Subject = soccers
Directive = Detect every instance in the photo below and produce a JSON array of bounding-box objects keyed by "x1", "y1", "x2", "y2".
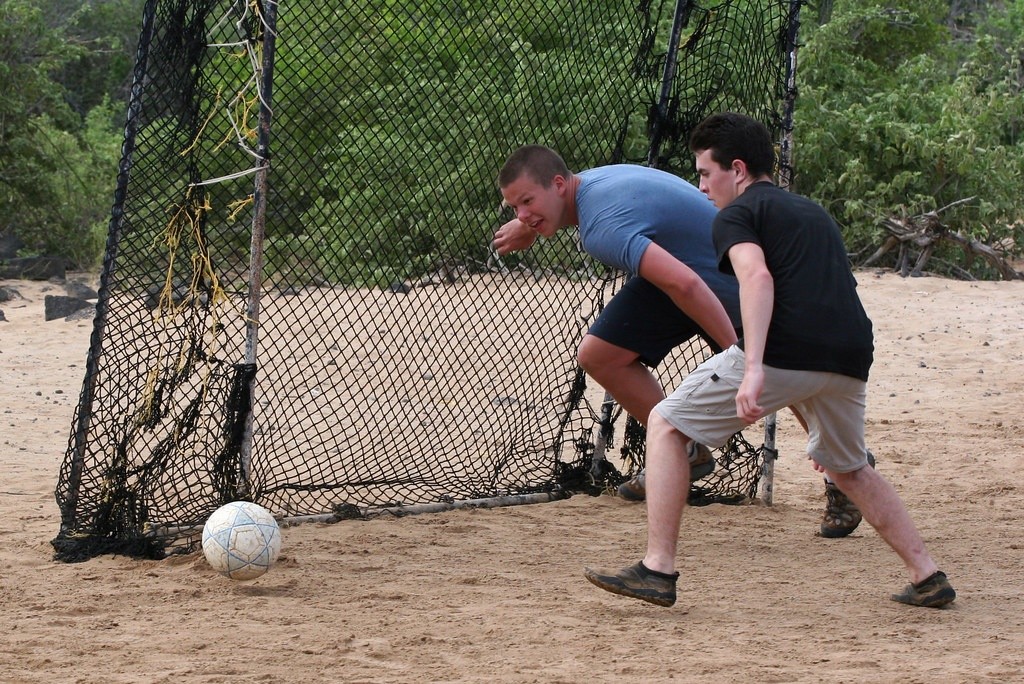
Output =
[{"x1": 201, "y1": 500, "x2": 282, "y2": 580}]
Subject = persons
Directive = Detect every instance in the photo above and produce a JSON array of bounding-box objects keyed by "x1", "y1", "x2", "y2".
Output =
[
  {"x1": 493, "y1": 143, "x2": 876, "y2": 538},
  {"x1": 581, "y1": 112, "x2": 956, "y2": 608}
]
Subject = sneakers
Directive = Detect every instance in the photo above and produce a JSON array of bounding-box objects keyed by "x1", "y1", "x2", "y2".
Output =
[
  {"x1": 618, "y1": 441, "x2": 715, "y2": 501},
  {"x1": 889, "y1": 570, "x2": 956, "y2": 606},
  {"x1": 584, "y1": 560, "x2": 680, "y2": 607},
  {"x1": 815, "y1": 450, "x2": 876, "y2": 541}
]
